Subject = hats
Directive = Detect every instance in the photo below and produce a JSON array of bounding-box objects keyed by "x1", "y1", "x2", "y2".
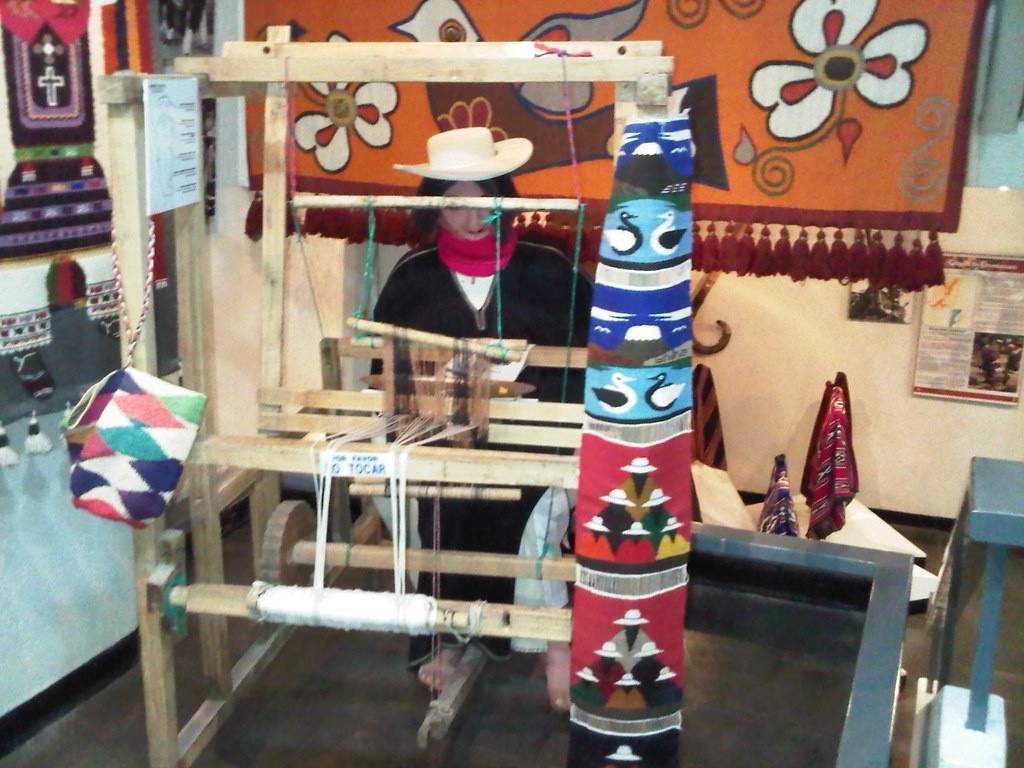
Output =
[{"x1": 392, "y1": 126, "x2": 534, "y2": 183}]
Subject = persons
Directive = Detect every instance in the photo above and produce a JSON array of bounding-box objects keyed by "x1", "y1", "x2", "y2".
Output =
[{"x1": 366, "y1": 128, "x2": 595, "y2": 712}]
